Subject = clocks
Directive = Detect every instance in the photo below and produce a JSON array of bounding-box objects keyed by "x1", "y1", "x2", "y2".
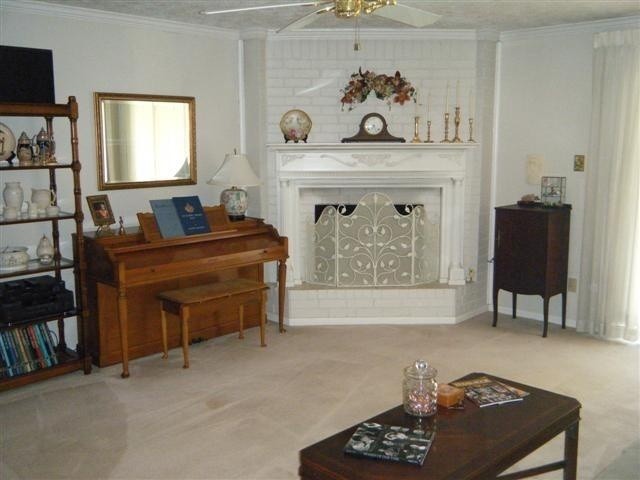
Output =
[{"x1": 341, "y1": 114, "x2": 405, "y2": 143}]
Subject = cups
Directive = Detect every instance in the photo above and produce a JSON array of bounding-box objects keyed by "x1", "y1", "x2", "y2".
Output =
[{"x1": 2, "y1": 201, "x2": 60, "y2": 219}]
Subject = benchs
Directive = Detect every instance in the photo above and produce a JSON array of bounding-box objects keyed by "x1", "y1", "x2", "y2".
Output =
[{"x1": 157, "y1": 279, "x2": 270, "y2": 368}]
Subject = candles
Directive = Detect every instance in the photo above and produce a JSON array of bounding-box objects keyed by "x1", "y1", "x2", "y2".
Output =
[{"x1": 414, "y1": 76, "x2": 475, "y2": 121}]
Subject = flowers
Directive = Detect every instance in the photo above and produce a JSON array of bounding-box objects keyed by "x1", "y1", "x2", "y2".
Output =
[{"x1": 341, "y1": 66, "x2": 416, "y2": 109}]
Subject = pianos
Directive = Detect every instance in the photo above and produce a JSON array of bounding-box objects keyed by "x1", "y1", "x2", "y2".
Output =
[{"x1": 72, "y1": 205, "x2": 288, "y2": 370}]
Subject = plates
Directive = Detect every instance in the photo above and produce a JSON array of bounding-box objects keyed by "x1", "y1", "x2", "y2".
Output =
[
  {"x1": 518, "y1": 200, "x2": 545, "y2": 207},
  {"x1": 281, "y1": 109, "x2": 313, "y2": 139},
  {"x1": 0, "y1": 123, "x2": 16, "y2": 161}
]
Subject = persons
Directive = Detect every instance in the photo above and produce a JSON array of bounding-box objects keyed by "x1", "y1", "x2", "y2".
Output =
[{"x1": 98, "y1": 204, "x2": 109, "y2": 219}]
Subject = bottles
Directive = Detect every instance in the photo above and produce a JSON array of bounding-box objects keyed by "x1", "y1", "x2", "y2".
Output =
[
  {"x1": 402, "y1": 360, "x2": 440, "y2": 417},
  {"x1": 36, "y1": 234, "x2": 55, "y2": 264},
  {"x1": 17, "y1": 131, "x2": 33, "y2": 163}
]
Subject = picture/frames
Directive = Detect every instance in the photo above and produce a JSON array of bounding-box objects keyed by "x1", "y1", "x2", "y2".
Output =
[{"x1": 86, "y1": 195, "x2": 116, "y2": 226}]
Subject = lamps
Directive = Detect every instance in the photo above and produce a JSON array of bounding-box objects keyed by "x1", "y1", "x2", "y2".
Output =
[{"x1": 207, "y1": 149, "x2": 261, "y2": 221}]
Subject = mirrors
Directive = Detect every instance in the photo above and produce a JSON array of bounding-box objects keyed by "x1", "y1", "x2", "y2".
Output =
[{"x1": 93, "y1": 92, "x2": 196, "y2": 190}]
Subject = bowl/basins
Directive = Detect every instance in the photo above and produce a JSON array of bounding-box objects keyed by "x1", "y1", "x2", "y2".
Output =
[{"x1": 0, "y1": 246, "x2": 30, "y2": 271}]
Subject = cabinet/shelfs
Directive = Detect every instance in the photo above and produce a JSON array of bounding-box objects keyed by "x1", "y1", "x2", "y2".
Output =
[
  {"x1": 0, "y1": 96, "x2": 92, "y2": 392},
  {"x1": 492, "y1": 204, "x2": 572, "y2": 337}
]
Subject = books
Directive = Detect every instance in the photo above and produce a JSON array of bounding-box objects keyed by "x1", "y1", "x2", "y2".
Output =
[
  {"x1": 149, "y1": 199, "x2": 186, "y2": 239},
  {"x1": 0, "y1": 322, "x2": 58, "y2": 377},
  {"x1": 450, "y1": 376, "x2": 529, "y2": 408},
  {"x1": 344, "y1": 421, "x2": 435, "y2": 467},
  {"x1": 172, "y1": 196, "x2": 212, "y2": 235}
]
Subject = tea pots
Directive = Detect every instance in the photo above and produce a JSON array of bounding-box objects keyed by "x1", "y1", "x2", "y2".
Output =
[
  {"x1": 31, "y1": 127, "x2": 56, "y2": 163},
  {"x1": 30, "y1": 187, "x2": 56, "y2": 208}
]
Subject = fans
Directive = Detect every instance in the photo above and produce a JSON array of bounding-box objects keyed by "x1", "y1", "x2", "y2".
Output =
[{"x1": 200, "y1": 0, "x2": 443, "y2": 34}]
